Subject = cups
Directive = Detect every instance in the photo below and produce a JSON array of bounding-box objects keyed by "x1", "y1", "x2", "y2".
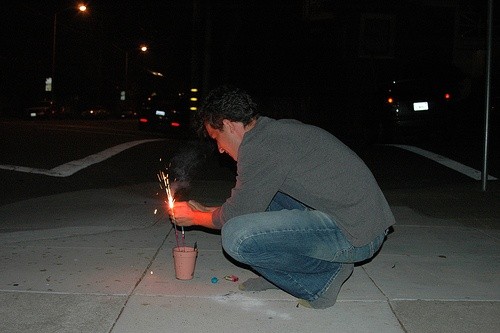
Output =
[{"x1": 173, "y1": 246, "x2": 198, "y2": 280}]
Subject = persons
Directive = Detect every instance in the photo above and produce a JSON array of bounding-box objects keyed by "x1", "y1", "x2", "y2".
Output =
[{"x1": 168, "y1": 85, "x2": 396, "y2": 309}]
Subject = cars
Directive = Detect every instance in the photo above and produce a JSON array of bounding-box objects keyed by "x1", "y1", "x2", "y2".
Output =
[
  {"x1": 384, "y1": 77, "x2": 457, "y2": 138},
  {"x1": 24, "y1": 98, "x2": 150, "y2": 122}
]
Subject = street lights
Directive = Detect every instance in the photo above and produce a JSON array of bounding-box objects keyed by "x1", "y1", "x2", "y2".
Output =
[
  {"x1": 124, "y1": 45, "x2": 147, "y2": 81},
  {"x1": 51, "y1": 6, "x2": 88, "y2": 119}
]
